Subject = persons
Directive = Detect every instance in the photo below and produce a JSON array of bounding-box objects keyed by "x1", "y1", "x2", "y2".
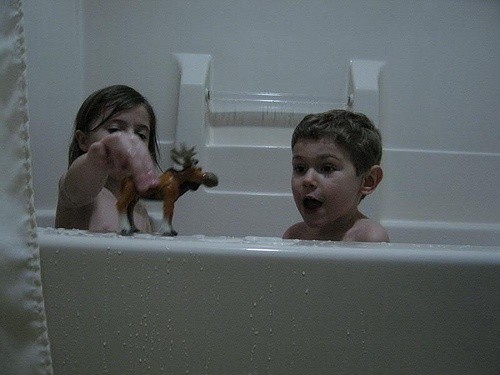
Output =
[
  {"x1": 56, "y1": 85, "x2": 165, "y2": 236},
  {"x1": 283, "y1": 109, "x2": 391, "y2": 243}
]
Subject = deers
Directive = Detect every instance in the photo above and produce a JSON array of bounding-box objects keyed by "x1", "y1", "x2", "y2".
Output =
[{"x1": 114, "y1": 145, "x2": 218, "y2": 237}]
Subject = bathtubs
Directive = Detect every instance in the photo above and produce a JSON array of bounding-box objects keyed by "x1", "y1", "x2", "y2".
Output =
[{"x1": 33, "y1": 140, "x2": 500, "y2": 375}]
